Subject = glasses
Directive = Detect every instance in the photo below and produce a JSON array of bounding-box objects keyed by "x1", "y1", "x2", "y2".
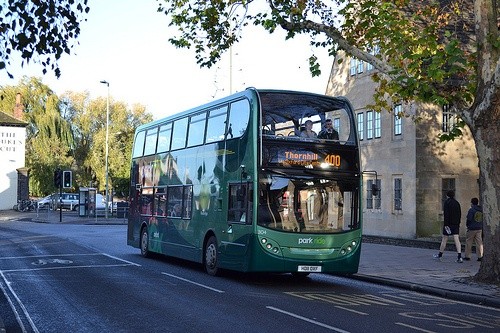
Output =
[{"x1": 326, "y1": 123, "x2": 332, "y2": 125}]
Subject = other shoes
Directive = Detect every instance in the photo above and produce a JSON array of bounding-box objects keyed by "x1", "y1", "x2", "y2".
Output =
[
  {"x1": 463, "y1": 257, "x2": 470, "y2": 262},
  {"x1": 477, "y1": 257, "x2": 483, "y2": 262}
]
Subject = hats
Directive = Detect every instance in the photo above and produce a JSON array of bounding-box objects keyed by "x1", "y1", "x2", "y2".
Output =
[
  {"x1": 471, "y1": 198, "x2": 478, "y2": 205},
  {"x1": 326, "y1": 119, "x2": 332, "y2": 122},
  {"x1": 447, "y1": 191, "x2": 455, "y2": 198}
]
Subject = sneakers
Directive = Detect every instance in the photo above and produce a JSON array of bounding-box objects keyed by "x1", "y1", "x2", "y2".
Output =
[
  {"x1": 457, "y1": 258, "x2": 464, "y2": 263},
  {"x1": 432, "y1": 253, "x2": 443, "y2": 260}
]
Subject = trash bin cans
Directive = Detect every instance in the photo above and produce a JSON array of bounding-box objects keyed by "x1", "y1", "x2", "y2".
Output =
[{"x1": 115, "y1": 200, "x2": 129, "y2": 217}]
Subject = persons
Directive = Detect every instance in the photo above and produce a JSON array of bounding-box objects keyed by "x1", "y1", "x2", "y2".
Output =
[
  {"x1": 318, "y1": 119, "x2": 340, "y2": 144},
  {"x1": 433, "y1": 190, "x2": 464, "y2": 263},
  {"x1": 300, "y1": 120, "x2": 317, "y2": 142},
  {"x1": 462, "y1": 198, "x2": 483, "y2": 261}
]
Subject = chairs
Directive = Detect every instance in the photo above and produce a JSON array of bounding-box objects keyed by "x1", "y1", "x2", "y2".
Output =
[{"x1": 262, "y1": 124, "x2": 300, "y2": 140}]
